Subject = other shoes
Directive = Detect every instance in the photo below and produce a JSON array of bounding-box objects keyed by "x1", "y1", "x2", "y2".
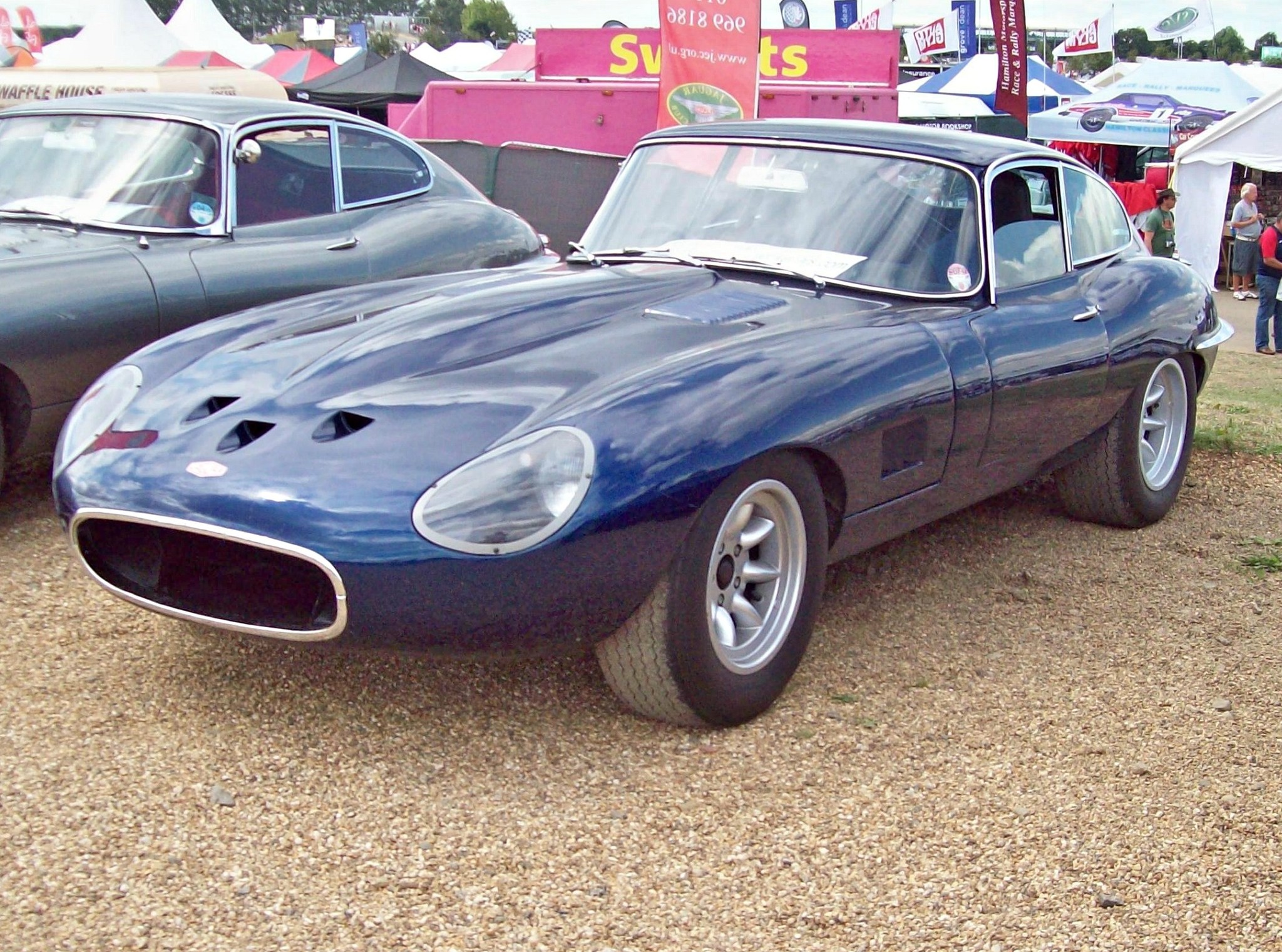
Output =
[
  {"x1": 1276, "y1": 350, "x2": 1282, "y2": 354},
  {"x1": 1259, "y1": 347, "x2": 1275, "y2": 355},
  {"x1": 1271, "y1": 333, "x2": 1275, "y2": 336}
]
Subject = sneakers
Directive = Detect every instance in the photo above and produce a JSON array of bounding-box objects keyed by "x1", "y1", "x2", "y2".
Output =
[
  {"x1": 1241, "y1": 290, "x2": 1259, "y2": 299},
  {"x1": 1233, "y1": 292, "x2": 1246, "y2": 300}
]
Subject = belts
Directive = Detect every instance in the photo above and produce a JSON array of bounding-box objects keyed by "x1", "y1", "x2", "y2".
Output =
[{"x1": 1236, "y1": 234, "x2": 1258, "y2": 241}]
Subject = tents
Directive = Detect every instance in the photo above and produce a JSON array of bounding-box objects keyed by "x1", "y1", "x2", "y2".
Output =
[
  {"x1": 3, "y1": 0, "x2": 534, "y2": 130},
  {"x1": 897, "y1": 51, "x2": 1282, "y2": 294}
]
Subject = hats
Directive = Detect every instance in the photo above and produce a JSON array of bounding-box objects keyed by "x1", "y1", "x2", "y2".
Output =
[{"x1": 1156, "y1": 188, "x2": 1180, "y2": 205}]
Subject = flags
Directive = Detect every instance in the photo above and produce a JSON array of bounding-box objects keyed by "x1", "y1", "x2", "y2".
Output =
[
  {"x1": 1052, "y1": 6, "x2": 1114, "y2": 57},
  {"x1": 834, "y1": 0, "x2": 858, "y2": 33},
  {"x1": 847, "y1": 0, "x2": 893, "y2": 33},
  {"x1": 779, "y1": 0, "x2": 811, "y2": 31},
  {"x1": 654, "y1": 0, "x2": 762, "y2": 132},
  {"x1": 989, "y1": 0, "x2": 1028, "y2": 127},
  {"x1": 952, "y1": 0, "x2": 977, "y2": 60},
  {"x1": 903, "y1": 8, "x2": 961, "y2": 64}
]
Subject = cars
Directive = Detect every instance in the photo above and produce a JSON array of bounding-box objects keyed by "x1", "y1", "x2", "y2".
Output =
[
  {"x1": 50, "y1": 116, "x2": 1234, "y2": 731},
  {"x1": 0, "y1": 94, "x2": 561, "y2": 496}
]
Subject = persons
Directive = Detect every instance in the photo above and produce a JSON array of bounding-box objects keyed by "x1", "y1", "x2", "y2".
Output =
[
  {"x1": 1144, "y1": 187, "x2": 1181, "y2": 258},
  {"x1": 1254, "y1": 212, "x2": 1282, "y2": 355},
  {"x1": 1230, "y1": 182, "x2": 1265, "y2": 301}
]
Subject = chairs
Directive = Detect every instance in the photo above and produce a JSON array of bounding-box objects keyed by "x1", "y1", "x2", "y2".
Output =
[{"x1": 905, "y1": 170, "x2": 1033, "y2": 284}]
